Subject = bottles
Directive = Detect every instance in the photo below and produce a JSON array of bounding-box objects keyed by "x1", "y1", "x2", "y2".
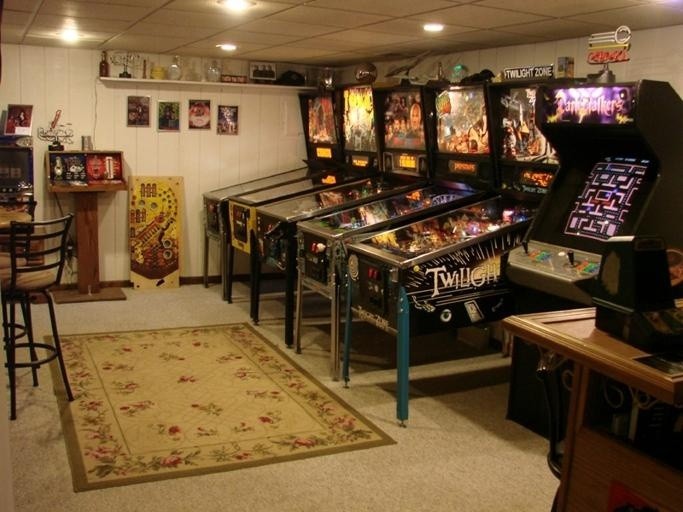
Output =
[
  {"x1": 208, "y1": 58, "x2": 222, "y2": 80},
  {"x1": 99, "y1": 50, "x2": 109, "y2": 77},
  {"x1": 167, "y1": 58, "x2": 182, "y2": 80}
]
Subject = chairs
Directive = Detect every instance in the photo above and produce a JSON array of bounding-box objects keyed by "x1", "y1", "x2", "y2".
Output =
[{"x1": 0, "y1": 199, "x2": 77, "y2": 422}]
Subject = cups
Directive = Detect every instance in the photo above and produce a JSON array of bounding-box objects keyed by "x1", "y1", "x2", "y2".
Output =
[{"x1": 81, "y1": 135, "x2": 94, "y2": 151}]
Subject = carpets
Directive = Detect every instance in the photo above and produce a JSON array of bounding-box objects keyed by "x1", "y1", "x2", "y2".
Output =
[{"x1": 42, "y1": 322, "x2": 398, "y2": 495}]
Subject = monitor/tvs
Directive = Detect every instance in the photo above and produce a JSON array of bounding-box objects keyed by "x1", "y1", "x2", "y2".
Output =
[{"x1": 561, "y1": 149, "x2": 652, "y2": 246}]
